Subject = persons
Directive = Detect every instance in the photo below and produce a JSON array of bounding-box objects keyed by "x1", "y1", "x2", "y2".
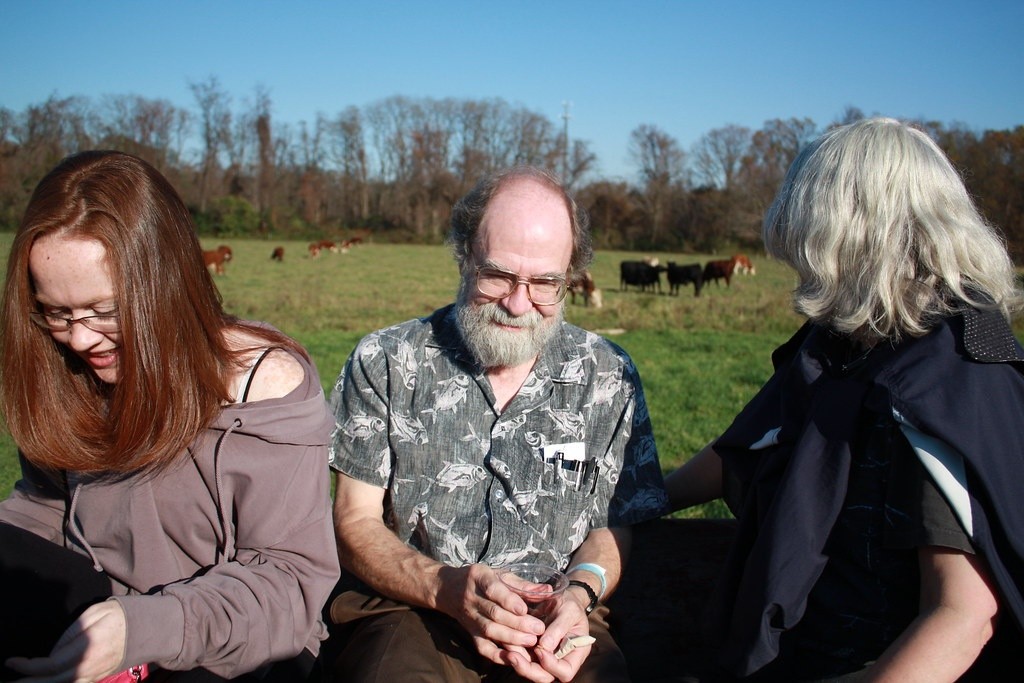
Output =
[
  {"x1": 326, "y1": 165, "x2": 669, "y2": 683},
  {"x1": 0, "y1": 149, "x2": 342, "y2": 683},
  {"x1": 663, "y1": 115, "x2": 1023, "y2": 683}
]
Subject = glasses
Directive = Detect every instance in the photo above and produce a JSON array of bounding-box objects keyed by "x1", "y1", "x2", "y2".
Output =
[
  {"x1": 30, "y1": 308, "x2": 125, "y2": 335},
  {"x1": 468, "y1": 248, "x2": 571, "y2": 306}
]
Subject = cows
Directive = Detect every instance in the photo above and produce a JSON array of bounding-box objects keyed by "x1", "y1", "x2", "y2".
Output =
[
  {"x1": 270, "y1": 245, "x2": 284, "y2": 261},
  {"x1": 308, "y1": 236, "x2": 365, "y2": 259},
  {"x1": 619, "y1": 253, "x2": 756, "y2": 298},
  {"x1": 565, "y1": 270, "x2": 602, "y2": 308},
  {"x1": 202, "y1": 245, "x2": 233, "y2": 276}
]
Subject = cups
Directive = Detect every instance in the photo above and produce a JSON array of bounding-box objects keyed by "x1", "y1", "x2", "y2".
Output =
[{"x1": 497, "y1": 562, "x2": 569, "y2": 629}]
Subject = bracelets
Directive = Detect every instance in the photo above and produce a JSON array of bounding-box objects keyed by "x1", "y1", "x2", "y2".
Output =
[
  {"x1": 569, "y1": 580, "x2": 598, "y2": 616},
  {"x1": 566, "y1": 563, "x2": 606, "y2": 601}
]
